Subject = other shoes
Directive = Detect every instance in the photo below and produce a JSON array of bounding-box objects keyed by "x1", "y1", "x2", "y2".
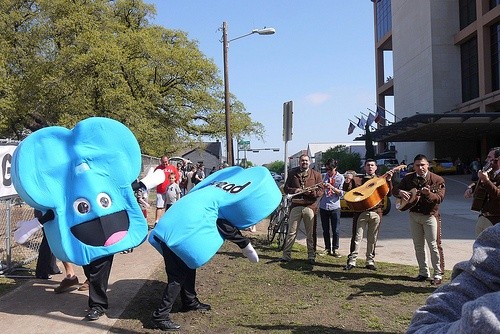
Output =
[
  {"x1": 152, "y1": 319, "x2": 182, "y2": 331},
  {"x1": 184, "y1": 302, "x2": 211, "y2": 312},
  {"x1": 431, "y1": 278, "x2": 441, "y2": 285},
  {"x1": 365, "y1": 265, "x2": 377, "y2": 271},
  {"x1": 54, "y1": 275, "x2": 79, "y2": 293},
  {"x1": 415, "y1": 275, "x2": 429, "y2": 281},
  {"x1": 319, "y1": 249, "x2": 331, "y2": 256},
  {"x1": 35, "y1": 273, "x2": 52, "y2": 280},
  {"x1": 333, "y1": 250, "x2": 342, "y2": 258},
  {"x1": 51, "y1": 270, "x2": 63, "y2": 275},
  {"x1": 78, "y1": 279, "x2": 90, "y2": 291},
  {"x1": 343, "y1": 264, "x2": 355, "y2": 270},
  {"x1": 85, "y1": 309, "x2": 105, "y2": 320}
]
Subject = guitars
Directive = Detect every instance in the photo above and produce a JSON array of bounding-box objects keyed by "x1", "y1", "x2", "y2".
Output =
[
  {"x1": 343, "y1": 163, "x2": 407, "y2": 212},
  {"x1": 395, "y1": 181, "x2": 446, "y2": 211}
]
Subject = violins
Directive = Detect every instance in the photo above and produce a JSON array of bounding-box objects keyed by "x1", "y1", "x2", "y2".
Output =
[{"x1": 480, "y1": 165, "x2": 495, "y2": 179}]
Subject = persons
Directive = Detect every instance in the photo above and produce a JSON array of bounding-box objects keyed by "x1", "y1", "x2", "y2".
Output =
[
  {"x1": 464, "y1": 147, "x2": 500, "y2": 239},
  {"x1": 14, "y1": 209, "x2": 115, "y2": 320},
  {"x1": 405, "y1": 222, "x2": 500, "y2": 334},
  {"x1": 280, "y1": 154, "x2": 324, "y2": 264},
  {"x1": 148, "y1": 219, "x2": 260, "y2": 331},
  {"x1": 455, "y1": 157, "x2": 463, "y2": 174},
  {"x1": 121, "y1": 155, "x2": 228, "y2": 255},
  {"x1": 342, "y1": 158, "x2": 393, "y2": 270},
  {"x1": 320, "y1": 158, "x2": 344, "y2": 258},
  {"x1": 392, "y1": 154, "x2": 446, "y2": 285}
]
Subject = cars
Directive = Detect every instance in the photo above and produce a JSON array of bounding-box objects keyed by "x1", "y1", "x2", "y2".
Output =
[
  {"x1": 338, "y1": 174, "x2": 392, "y2": 216},
  {"x1": 432, "y1": 159, "x2": 457, "y2": 175},
  {"x1": 168, "y1": 156, "x2": 192, "y2": 167},
  {"x1": 269, "y1": 170, "x2": 286, "y2": 183}
]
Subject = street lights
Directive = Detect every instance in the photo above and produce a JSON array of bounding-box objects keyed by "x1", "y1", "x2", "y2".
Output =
[
  {"x1": 216, "y1": 21, "x2": 276, "y2": 168},
  {"x1": 236, "y1": 148, "x2": 280, "y2": 166}
]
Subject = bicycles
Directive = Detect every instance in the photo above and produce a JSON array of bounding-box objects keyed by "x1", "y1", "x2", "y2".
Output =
[{"x1": 267, "y1": 193, "x2": 292, "y2": 252}]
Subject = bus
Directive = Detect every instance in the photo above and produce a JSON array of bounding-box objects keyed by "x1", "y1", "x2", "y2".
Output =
[{"x1": 313, "y1": 167, "x2": 329, "y2": 179}]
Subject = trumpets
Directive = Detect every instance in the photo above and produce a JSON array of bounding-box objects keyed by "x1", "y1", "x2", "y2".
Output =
[{"x1": 285, "y1": 180, "x2": 331, "y2": 201}]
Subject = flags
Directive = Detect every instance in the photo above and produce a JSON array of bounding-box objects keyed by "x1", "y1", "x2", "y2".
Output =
[
  {"x1": 357, "y1": 106, "x2": 382, "y2": 129},
  {"x1": 348, "y1": 122, "x2": 355, "y2": 135}
]
